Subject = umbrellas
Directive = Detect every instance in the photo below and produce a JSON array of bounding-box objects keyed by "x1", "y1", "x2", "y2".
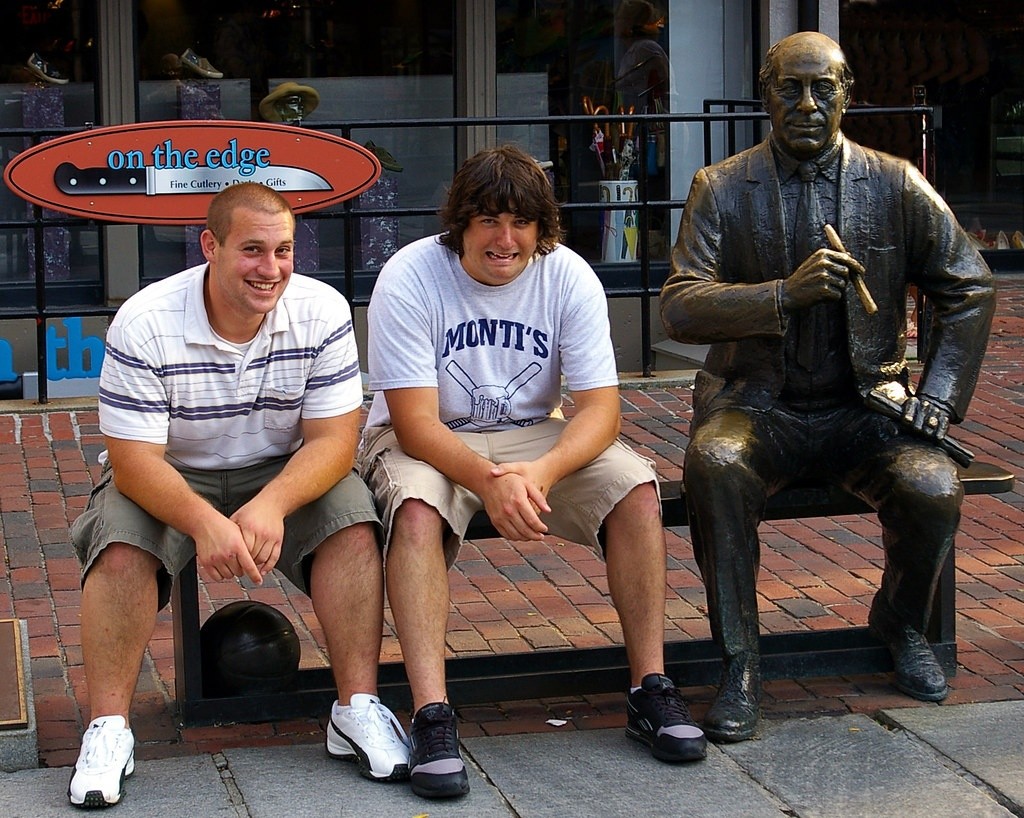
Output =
[{"x1": 580, "y1": 97, "x2": 667, "y2": 179}]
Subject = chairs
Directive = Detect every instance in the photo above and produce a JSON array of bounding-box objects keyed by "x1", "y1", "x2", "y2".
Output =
[{"x1": 988, "y1": 88, "x2": 1024, "y2": 193}]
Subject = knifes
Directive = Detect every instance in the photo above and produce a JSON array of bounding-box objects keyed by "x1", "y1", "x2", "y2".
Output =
[{"x1": 53, "y1": 162, "x2": 334, "y2": 195}]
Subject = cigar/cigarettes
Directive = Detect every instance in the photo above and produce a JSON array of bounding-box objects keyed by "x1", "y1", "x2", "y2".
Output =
[{"x1": 823, "y1": 223, "x2": 878, "y2": 315}]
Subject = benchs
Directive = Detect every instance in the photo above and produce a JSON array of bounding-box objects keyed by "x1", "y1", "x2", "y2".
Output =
[{"x1": 171, "y1": 462, "x2": 1016, "y2": 728}]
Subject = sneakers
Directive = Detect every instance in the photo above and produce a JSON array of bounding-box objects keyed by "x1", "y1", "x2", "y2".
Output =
[
  {"x1": 67, "y1": 714, "x2": 135, "y2": 809},
  {"x1": 626, "y1": 673, "x2": 707, "y2": 764},
  {"x1": 28, "y1": 53, "x2": 69, "y2": 85},
  {"x1": 407, "y1": 695, "x2": 470, "y2": 799},
  {"x1": 324, "y1": 693, "x2": 413, "y2": 784},
  {"x1": 364, "y1": 139, "x2": 403, "y2": 173}
]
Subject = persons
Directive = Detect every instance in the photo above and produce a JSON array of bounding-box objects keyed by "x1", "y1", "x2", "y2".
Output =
[
  {"x1": 662, "y1": 30, "x2": 997, "y2": 745},
  {"x1": 353, "y1": 144, "x2": 706, "y2": 798},
  {"x1": 61, "y1": 183, "x2": 414, "y2": 807},
  {"x1": 614, "y1": 1, "x2": 669, "y2": 127}
]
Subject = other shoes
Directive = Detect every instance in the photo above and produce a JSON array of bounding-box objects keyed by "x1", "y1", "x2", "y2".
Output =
[
  {"x1": 181, "y1": 49, "x2": 224, "y2": 79},
  {"x1": 965, "y1": 229, "x2": 1023, "y2": 250},
  {"x1": 160, "y1": 53, "x2": 181, "y2": 68}
]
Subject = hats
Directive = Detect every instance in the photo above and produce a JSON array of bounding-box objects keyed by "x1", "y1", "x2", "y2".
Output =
[{"x1": 260, "y1": 82, "x2": 320, "y2": 122}]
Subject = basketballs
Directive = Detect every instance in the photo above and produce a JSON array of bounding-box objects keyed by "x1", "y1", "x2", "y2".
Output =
[{"x1": 198, "y1": 598, "x2": 302, "y2": 698}]
